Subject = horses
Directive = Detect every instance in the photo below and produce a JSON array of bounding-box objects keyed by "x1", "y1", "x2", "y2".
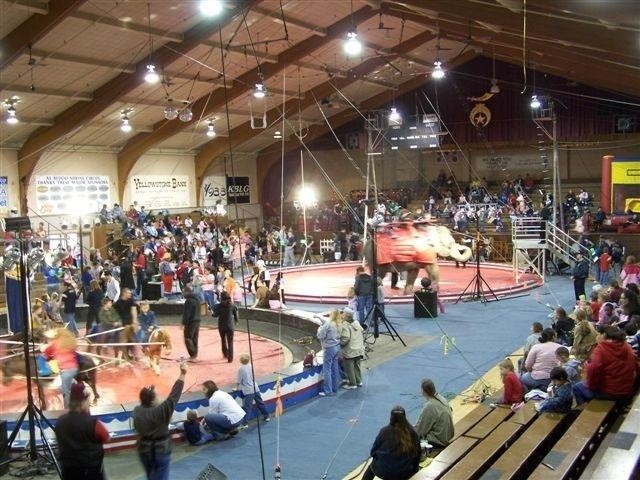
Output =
[
  {"x1": 0, "y1": 351, "x2": 100, "y2": 407},
  {"x1": 84, "y1": 324, "x2": 124, "y2": 368},
  {"x1": 113, "y1": 324, "x2": 172, "y2": 377}
]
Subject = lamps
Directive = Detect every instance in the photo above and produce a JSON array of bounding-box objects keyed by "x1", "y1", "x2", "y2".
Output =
[
  {"x1": 432, "y1": 61, "x2": 445, "y2": 79},
  {"x1": 3, "y1": 96, "x2": 21, "y2": 125},
  {"x1": 145, "y1": 52, "x2": 160, "y2": 86},
  {"x1": 253, "y1": 80, "x2": 266, "y2": 99},
  {"x1": 344, "y1": 31, "x2": 362, "y2": 57},
  {"x1": 274, "y1": 131, "x2": 282, "y2": 138},
  {"x1": 200, "y1": 1, "x2": 222, "y2": 17},
  {"x1": 530, "y1": 94, "x2": 541, "y2": 109},
  {"x1": 389, "y1": 105, "x2": 402, "y2": 123},
  {"x1": 207, "y1": 120, "x2": 217, "y2": 138},
  {"x1": 121, "y1": 110, "x2": 133, "y2": 133}
]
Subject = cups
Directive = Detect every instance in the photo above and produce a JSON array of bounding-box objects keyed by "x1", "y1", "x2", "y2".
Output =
[
  {"x1": 579, "y1": 295, "x2": 585, "y2": 301},
  {"x1": 426, "y1": 445, "x2": 432, "y2": 457}
]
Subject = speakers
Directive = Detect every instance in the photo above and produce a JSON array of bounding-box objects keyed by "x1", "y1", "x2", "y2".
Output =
[
  {"x1": 414, "y1": 291, "x2": 438, "y2": 318},
  {"x1": 193, "y1": 462, "x2": 228, "y2": 480},
  {"x1": 0, "y1": 419, "x2": 9, "y2": 477}
]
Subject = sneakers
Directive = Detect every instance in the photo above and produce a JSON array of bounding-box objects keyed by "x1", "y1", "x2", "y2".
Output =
[{"x1": 343, "y1": 382, "x2": 363, "y2": 389}]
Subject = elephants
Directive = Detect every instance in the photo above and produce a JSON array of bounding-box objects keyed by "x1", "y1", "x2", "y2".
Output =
[{"x1": 362, "y1": 225, "x2": 473, "y2": 296}]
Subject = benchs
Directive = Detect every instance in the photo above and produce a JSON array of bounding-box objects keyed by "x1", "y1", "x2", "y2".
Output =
[
  {"x1": 412, "y1": 376, "x2": 640, "y2": 480},
  {"x1": 1, "y1": 238, "x2": 82, "y2": 309},
  {"x1": 94, "y1": 209, "x2": 228, "y2": 259},
  {"x1": 406, "y1": 181, "x2": 602, "y2": 234}
]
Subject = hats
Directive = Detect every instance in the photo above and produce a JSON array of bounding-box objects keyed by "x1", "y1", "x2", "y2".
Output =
[{"x1": 71, "y1": 377, "x2": 93, "y2": 400}]
